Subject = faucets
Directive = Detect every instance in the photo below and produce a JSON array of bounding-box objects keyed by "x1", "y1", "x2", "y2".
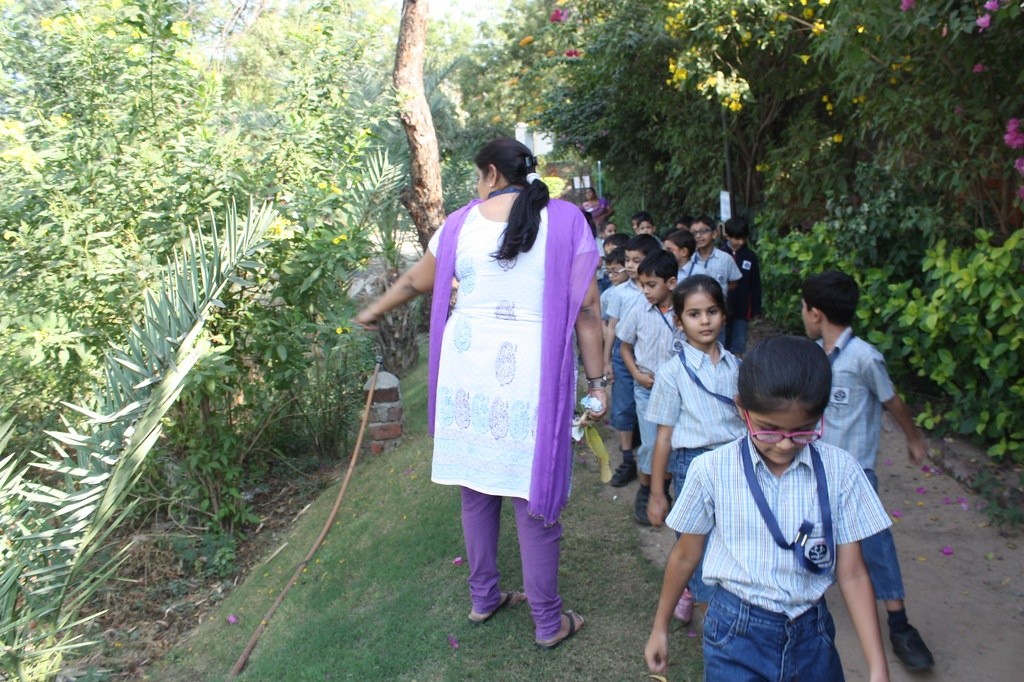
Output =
[{"x1": 370, "y1": 347, "x2": 383, "y2": 364}]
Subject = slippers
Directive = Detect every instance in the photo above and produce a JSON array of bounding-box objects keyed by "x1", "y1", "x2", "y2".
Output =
[
  {"x1": 536, "y1": 612, "x2": 585, "y2": 648},
  {"x1": 467, "y1": 591, "x2": 526, "y2": 624}
]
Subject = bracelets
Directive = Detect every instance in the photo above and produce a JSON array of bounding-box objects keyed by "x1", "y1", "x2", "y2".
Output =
[
  {"x1": 588, "y1": 375, "x2": 607, "y2": 388},
  {"x1": 587, "y1": 373, "x2": 605, "y2": 380}
]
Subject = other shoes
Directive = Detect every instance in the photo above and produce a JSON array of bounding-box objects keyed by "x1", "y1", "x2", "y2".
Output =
[
  {"x1": 634, "y1": 489, "x2": 652, "y2": 523},
  {"x1": 890, "y1": 625, "x2": 934, "y2": 671}
]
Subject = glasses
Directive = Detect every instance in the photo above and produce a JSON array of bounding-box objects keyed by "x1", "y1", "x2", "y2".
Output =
[
  {"x1": 605, "y1": 268, "x2": 626, "y2": 275},
  {"x1": 741, "y1": 406, "x2": 825, "y2": 445},
  {"x1": 691, "y1": 227, "x2": 710, "y2": 236}
]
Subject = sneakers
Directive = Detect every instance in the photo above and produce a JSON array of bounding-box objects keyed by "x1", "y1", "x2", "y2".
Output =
[
  {"x1": 611, "y1": 460, "x2": 637, "y2": 487},
  {"x1": 673, "y1": 588, "x2": 696, "y2": 623}
]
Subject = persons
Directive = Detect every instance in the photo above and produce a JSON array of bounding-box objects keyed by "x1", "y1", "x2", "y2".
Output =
[
  {"x1": 580, "y1": 185, "x2": 764, "y2": 624},
  {"x1": 799, "y1": 271, "x2": 936, "y2": 674},
  {"x1": 644, "y1": 338, "x2": 894, "y2": 682},
  {"x1": 355, "y1": 138, "x2": 609, "y2": 648}
]
